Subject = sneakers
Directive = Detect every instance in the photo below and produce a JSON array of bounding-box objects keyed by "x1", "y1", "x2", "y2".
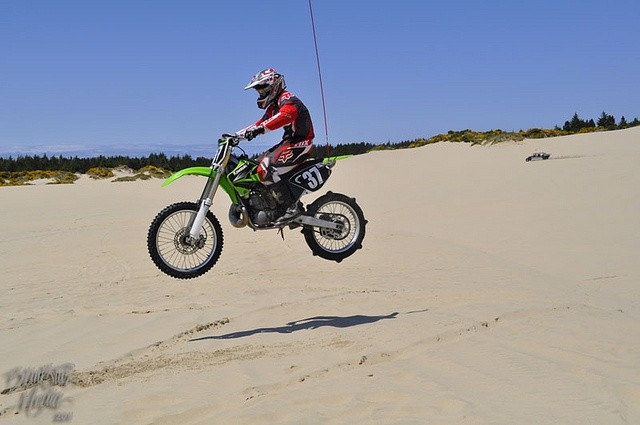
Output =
[{"x1": 277, "y1": 205, "x2": 302, "y2": 222}]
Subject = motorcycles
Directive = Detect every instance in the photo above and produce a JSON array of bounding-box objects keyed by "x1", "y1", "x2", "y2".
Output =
[{"x1": 147, "y1": 131, "x2": 369, "y2": 280}]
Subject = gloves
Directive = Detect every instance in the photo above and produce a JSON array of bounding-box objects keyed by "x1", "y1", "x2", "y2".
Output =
[{"x1": 245, "y1": 126, "x2": 265, "y2": 142}]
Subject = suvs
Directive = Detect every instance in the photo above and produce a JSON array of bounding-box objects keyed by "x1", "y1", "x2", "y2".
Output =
[{"x1": 525, "y1": 152, "x2": 551, "y2": 162}]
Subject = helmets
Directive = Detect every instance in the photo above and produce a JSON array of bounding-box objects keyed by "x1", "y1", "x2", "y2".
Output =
[{"x1": 244, "y1": 68, "x2": 287, "y2": 109}]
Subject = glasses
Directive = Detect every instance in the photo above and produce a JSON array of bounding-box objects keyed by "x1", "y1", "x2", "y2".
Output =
[{"x1": 257, "y1": 75, "x2": 283, "y2": 96}]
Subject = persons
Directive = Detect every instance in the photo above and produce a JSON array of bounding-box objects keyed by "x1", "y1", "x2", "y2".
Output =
[{"x1": 234, "y1": 68, "x2": 315, "y2": 225}]
